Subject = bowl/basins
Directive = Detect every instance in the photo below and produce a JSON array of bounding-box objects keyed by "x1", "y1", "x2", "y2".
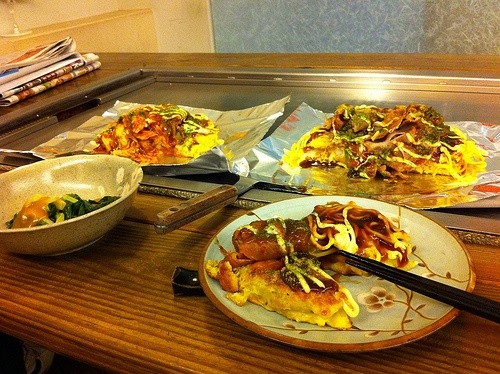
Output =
[{"x1": 0, "y1": 154, "x2": 142, "y2": 258}]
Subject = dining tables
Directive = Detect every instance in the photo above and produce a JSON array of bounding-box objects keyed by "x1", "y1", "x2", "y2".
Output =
[{"x1": 0, "y1": 50, "x2": 500, "y2": 374}]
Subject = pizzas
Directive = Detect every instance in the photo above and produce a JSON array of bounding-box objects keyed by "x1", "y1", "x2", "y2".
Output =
[
  {"x1": 281, "y1": 102, "x2": 488, "y2": 184},
  {"x1": 206, "y1": 200, "x2": 417, "y2": 328},
  {"x1": 93, "y1": 103, "x2": 226, "y2": 166}
]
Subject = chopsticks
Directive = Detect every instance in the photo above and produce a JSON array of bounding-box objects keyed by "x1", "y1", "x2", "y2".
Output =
[{"x1": 337, "y1": 250, "x2": 499, "y2": 328}]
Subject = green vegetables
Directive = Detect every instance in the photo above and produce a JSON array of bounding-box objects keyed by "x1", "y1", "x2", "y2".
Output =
[{"x1": 7, "y1": 194, "x2": 121, "y2": 229}]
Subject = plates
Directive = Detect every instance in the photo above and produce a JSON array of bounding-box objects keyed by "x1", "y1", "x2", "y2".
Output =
[{"x1": 198, "y1": 198, "x2": 478, "y2": 353}]
